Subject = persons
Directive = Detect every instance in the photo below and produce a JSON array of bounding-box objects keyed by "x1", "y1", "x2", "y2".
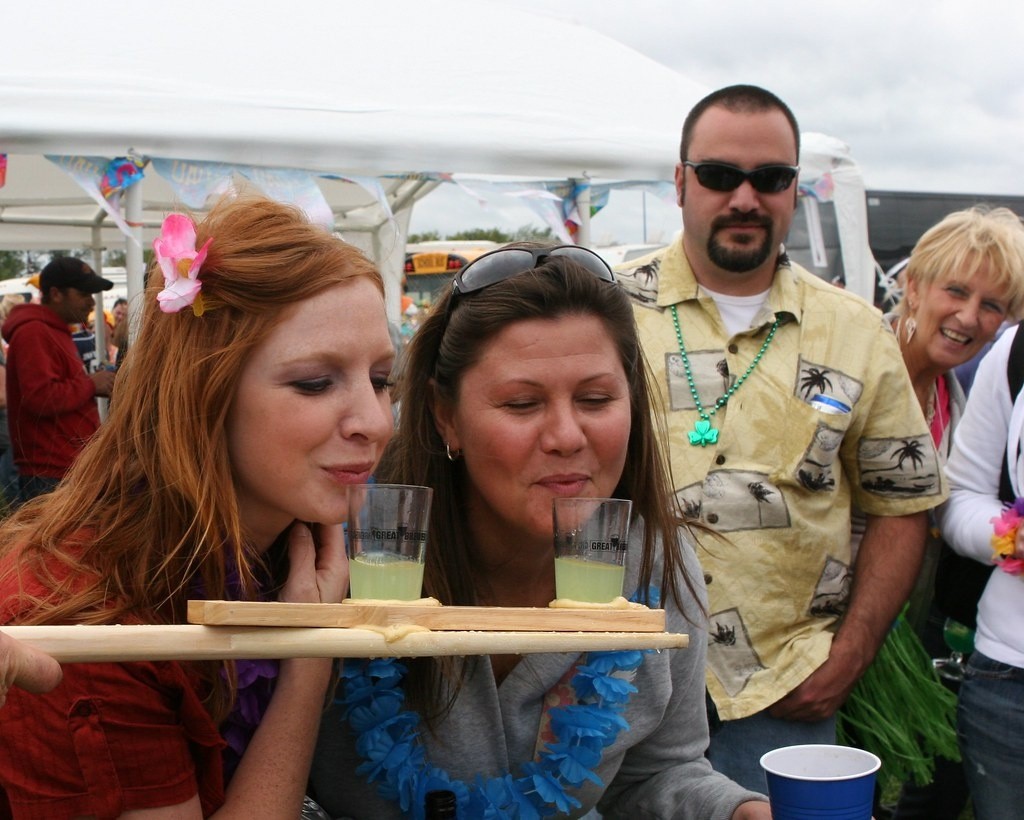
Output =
[
  {"x1": 0, "y1": 83, "x2": 1024, "y2": 820},
  {"x1": 1, "y1": 189, "x2": 396, "y2": 820},
  {"x1": 304, "y1": 240, "x2": 774, "y2": 820}
]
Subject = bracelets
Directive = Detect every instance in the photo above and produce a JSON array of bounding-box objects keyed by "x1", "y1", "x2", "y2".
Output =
[{"x1": 988, "y1": 497, "x2": 1024, "y2": 576}]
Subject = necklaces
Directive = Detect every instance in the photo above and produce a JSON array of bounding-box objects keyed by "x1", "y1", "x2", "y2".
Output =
[
  {"x1": 897, "y1": 318, "x2": 949, "y2": 460},
  {"x1": 670, "y1": 303, "x2": 780, "y2": 448},
  {"x1": 337, "y1": 472, "x2": 661, "y2": 820},
  {"x1": 224, "y1": 659, "x2": 279, "y2": 778}
]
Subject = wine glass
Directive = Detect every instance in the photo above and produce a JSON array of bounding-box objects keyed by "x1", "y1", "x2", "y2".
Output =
[{"x1": 932, "y1": 616, "x2": 975, "y2": 680}]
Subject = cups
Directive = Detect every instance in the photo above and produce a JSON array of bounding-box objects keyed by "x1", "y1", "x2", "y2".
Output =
[
  {"x1": 552, "y1": 497, "x2": 633, "y2": 603},
  {"x1": 346, "y1": 483, "x2": 434, "y2": 601},
  {"x1": 94, "y1": 361, "x2": 117, "y2": 372},
  {"x1": 760, "y1": 744, "x2": 882, "y2": 820}
]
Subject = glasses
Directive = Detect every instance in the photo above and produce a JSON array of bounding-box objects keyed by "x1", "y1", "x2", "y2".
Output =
[
  {"x1": 444, "y1": 245, "x2": 618, "y2": 320},
  {"x1": 684, "y1": 160, "x2": 800, "y2": 192}
]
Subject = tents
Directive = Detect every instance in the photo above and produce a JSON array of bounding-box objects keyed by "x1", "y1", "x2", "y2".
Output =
[{"x1": 1, "y1": 17, "x2": 1024, "y2": 425}]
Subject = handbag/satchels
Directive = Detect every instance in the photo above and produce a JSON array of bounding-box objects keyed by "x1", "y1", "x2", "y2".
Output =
[{"x1": 930, "y1": 497, "x2": 1016, "y2": 631}]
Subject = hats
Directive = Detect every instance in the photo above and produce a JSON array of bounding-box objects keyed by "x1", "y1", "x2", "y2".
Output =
[{"x1": 40, "y1": 258, "x2": 114, "y2": 293}]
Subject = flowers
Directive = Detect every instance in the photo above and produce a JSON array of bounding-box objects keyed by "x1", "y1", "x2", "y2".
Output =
[{"x1": 144, "y1": 211, "x2": 214, "y2": 314}]
